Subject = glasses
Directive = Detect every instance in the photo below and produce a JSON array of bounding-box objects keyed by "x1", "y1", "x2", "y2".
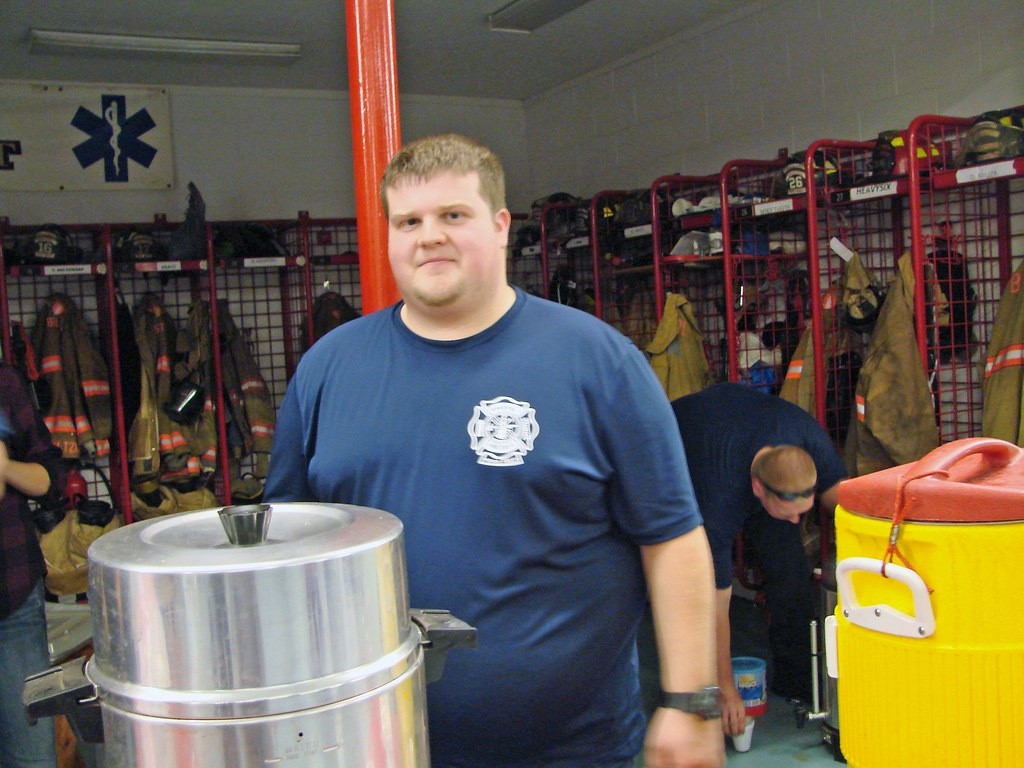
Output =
[{"x1": 754, "y1": 474, "x2": 818, "y2": 500}]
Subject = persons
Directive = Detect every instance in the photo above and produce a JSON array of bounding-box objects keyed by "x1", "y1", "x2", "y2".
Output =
[
  {"x1": 0, "y1": 365, "x2": 64, "y2": 767},
  {"x1": 263, "y1": 132, "x2": 730, "y2": 768},
  {"x1": 669, "y1": 382, "x2": 848, "y2": 737}
]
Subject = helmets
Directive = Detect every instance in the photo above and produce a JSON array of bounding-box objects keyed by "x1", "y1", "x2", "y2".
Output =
[
  {"x1": 781, "y1": 149, "x2": 840, "y2": 195},
  {"x1": 959, "y1": 111, "x2": 1024, "y2": 168},
  {"x1": 866, "y1": 130, "x2": 942, "y2": 183}
]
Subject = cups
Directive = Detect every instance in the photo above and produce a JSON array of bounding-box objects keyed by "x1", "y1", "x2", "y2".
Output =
[{"x1": 732, "y1": 719, "x2": 755, "y2": 752}]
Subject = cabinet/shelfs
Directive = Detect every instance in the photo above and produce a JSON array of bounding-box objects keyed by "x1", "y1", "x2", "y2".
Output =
[{"x1": 0, "y1": 105, "x2": 1024, "y2": 585}]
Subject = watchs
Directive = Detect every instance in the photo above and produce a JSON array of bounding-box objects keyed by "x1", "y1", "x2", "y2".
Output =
[{"x1": 658, "y1": 685, "x2": 728, "y2": 720}]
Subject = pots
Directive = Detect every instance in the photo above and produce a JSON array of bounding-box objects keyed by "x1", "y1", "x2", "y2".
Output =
[{"x1": 22, "y1": 500, "x2": 480, "y2": 768}]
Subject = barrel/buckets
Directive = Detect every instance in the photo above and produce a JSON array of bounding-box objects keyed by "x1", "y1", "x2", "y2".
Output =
[
  {"x1": 824, "y1": 437, "x2": 1024, "y2": 768},
  {"x1": 731, "y1": 657, "x2": 768, "y2": 717}
]
